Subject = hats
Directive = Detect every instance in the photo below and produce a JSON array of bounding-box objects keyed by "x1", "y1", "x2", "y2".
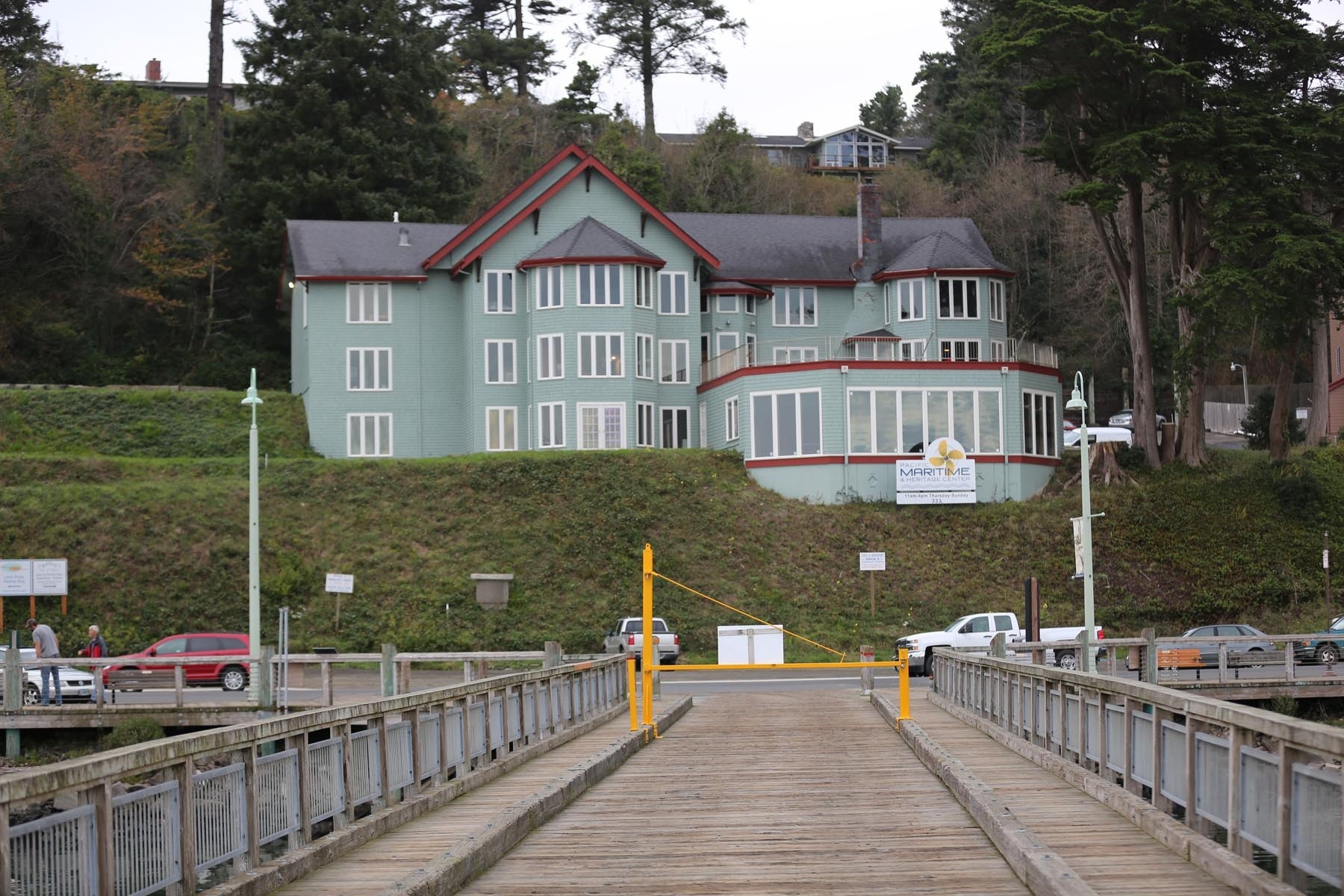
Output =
[{"x1": 25, "y1": 618, "x2": 36, "y2": 628}]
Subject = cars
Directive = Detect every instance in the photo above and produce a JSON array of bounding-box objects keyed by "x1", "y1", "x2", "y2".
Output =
[
  {"x1": 1285, "y1": 615, "x2": 1344, "y2": 665},
  {"x1": 1063, "y1": 417, "x2": 1135, "y2": 453},
  {"x1": 0, "y1": 647, "x2": 96, "y2": 708},
  {"x1": 1108, "y1": 409, "x2": 1167, "y2": 433},
  {"x1": 1125, "y1": 625, "x2": 1276, "y2": 669}
]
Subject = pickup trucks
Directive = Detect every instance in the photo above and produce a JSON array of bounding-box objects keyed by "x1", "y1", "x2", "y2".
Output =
[
  {"x1": 892, "y1": 613, "x2": 1106, "y2": 680},
  {"x1": 602, "y1": 618, "x2": 681, "y2": 674}
]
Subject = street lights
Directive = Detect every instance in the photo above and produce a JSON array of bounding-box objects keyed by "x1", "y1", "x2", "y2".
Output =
[
  {"x1": 1230, "y1": 361, "x2": 1250, "y2": 426},
  {"x1": 240, "y1": 365, "x2": 265, "y2": 712},
  {"x1": 1065, "y1": 368, "x2": 1103, "y2": 679}
]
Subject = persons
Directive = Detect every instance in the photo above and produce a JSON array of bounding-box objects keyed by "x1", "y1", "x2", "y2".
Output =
[
  {"x1": 26, "y1": 618, "x2": 63, "y2": 706},
  {"x1": 78, "y1": 626, "x2": 108, "y2": 704}
]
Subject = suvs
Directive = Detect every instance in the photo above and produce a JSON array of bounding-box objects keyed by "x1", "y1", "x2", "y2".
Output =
[{"x1": 100, "y1": 633, "x2": 252, "y2": 692}]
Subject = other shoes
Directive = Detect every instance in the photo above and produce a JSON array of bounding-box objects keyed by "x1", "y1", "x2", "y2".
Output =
[
  {"x1": 104, "y1": 701, "x2": 107, "y2": 703},
  {"x1": 36, "y1": 704, "x2": 49, "y2": 707},
  {"x1": 56, "y1": 705, "x2": 61, "y2": 706},
  {"x1": 87, "y1": 700, "x2": 97, "y2": 703}
]
